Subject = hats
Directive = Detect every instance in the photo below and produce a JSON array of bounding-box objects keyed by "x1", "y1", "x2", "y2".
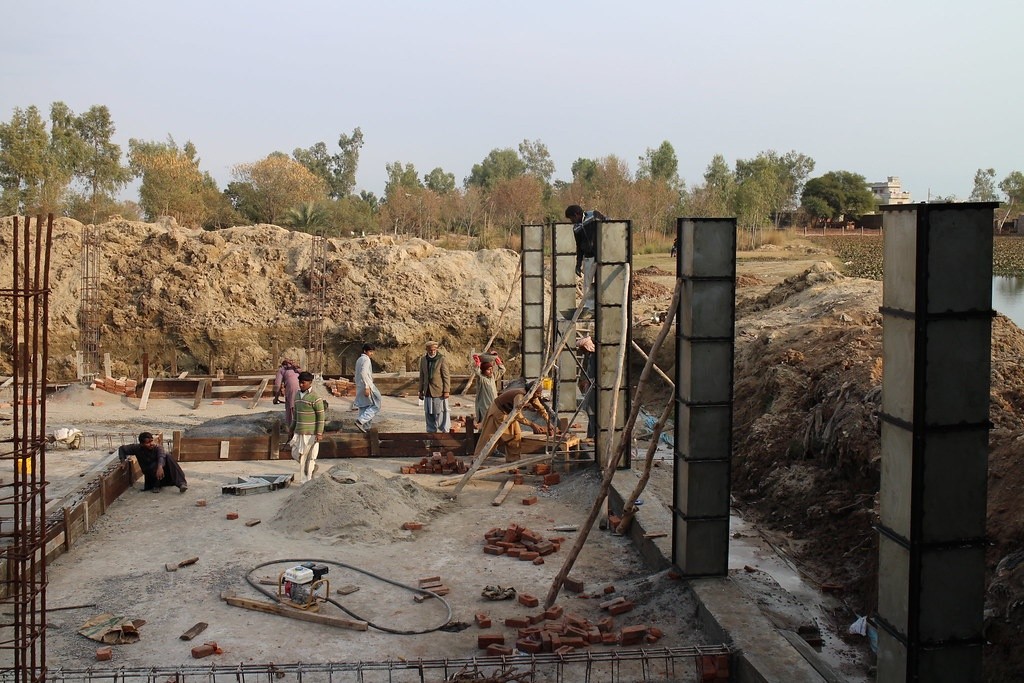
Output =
[
  {"x1": 529, "y1": 380, "x2": 543, "y2": 392},
  {"x1": 480, "y1": 362, "x2": 492, "y2": 372},
  {"x1": 576, "y1": 336, "x2": 595, "y2": 352}
]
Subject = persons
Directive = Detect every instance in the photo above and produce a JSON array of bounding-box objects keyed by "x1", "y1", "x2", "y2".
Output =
[
  {"x1": 564, "y1": 203, "x2": 608, "y2": 321},
  {"x1": 287, "y1": 372, "x2": 325, "y2": 485},
  {"x1": 119, "y1": 432, "x2": 188, "y2": 492},
  {"x1": 577, "y1": 336, "x2": 596, "y2": 439},
  {"x1": 473, "y1": 350, "x2": 507, "y2": 458},
  {"x1": 671, "y1": 238, "x2": 677, "y2": 257},
  {"x1": 352, "y1": 343, "x2": 382, "y2": 434},
  {"x1": 470, "y1": 380, "x2": 555, "y2": 470},
  {"x1": 419, "y1": 340, "x2": 451, "y2": 448},
  {"x1": 272, "y1": 361, "x2": 300, "y2": 438}
]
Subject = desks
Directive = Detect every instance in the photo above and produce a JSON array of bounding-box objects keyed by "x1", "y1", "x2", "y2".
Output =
[{"x1": 520, "y1": 429, "x2": 580, "y2": 475}]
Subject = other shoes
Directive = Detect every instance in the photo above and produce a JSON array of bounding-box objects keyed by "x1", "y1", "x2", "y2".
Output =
[
  {"x1": 304, "y1": 465, "x2": 318, "y2": 475},
  {"x1": 518, "y1": 464, "x2": 527, "y2": 470},
  {"x1": 491, "y1": 450, "x2": 505, "y2": 457},
  {"x1": 180, "y1": 484, "x2": 188, "y2": 492},
  {"x1": 354, "y1": 420, "x2": 367, "y2": 433},
  {"x1": 152, "y1": 486, "x2": 161, "y2": 492}
]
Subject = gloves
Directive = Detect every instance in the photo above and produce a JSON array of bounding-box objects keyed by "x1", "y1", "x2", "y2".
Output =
[
  {"x1": 472, "y1": 354, "x2": 480, "y2": 368},
  {"x1": 491, "y1": 350, "x2": 502, "y2": 365}
]
